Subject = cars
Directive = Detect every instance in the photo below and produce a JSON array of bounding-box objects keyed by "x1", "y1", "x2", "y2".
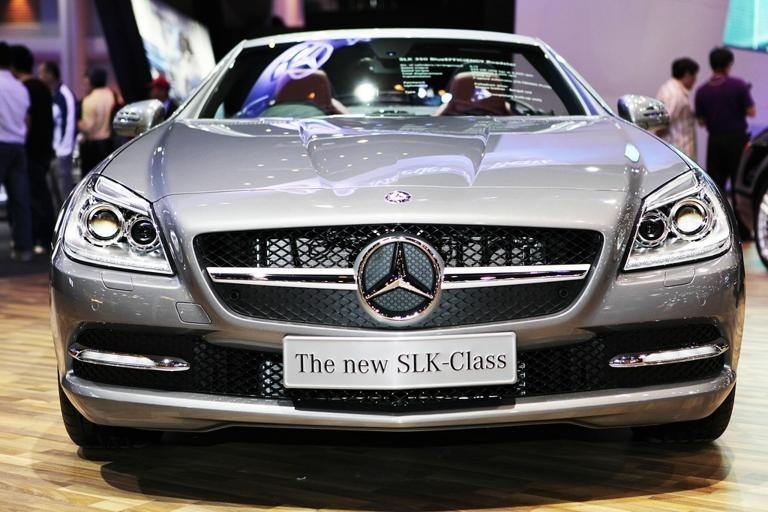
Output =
[{"x1": 733, "y1": 124, "x2": 767, "y2": 268}]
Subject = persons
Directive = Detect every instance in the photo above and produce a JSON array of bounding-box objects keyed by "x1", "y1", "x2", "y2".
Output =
[
  {"x1": 694, "y1": 47, "x2": 756, "y2": 241},
  {"x1": 656, "y1": 56, "x2": 698, "y2": 164}
]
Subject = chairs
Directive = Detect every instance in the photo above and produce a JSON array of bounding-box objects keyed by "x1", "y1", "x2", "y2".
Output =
[
  {"x1": 272, "y1": 68, "x2": 348, "y2": 118},
  {"x1": 429, "y1": 69, "x2": 516, "y2": 123}
]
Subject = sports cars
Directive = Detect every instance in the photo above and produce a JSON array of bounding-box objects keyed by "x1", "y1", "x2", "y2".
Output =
[{"x1": 48, "y1": 27, "x2": 747, "y2": 462}]
